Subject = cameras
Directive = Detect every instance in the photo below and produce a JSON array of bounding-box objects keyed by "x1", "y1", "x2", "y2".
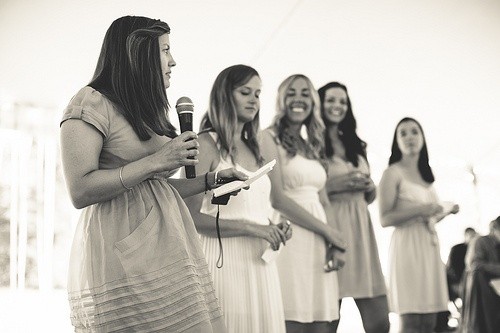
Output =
[{"x1": 210, "y1": 179, "x2": 230, "y2": 205}]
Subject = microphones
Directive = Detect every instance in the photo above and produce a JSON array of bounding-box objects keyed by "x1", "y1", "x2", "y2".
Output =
[{"x1": 175, "y1": 97, "x2": 196, "y2": 179}]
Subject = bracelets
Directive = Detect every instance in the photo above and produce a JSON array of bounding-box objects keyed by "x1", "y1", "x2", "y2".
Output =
[
  {"x1": 204, "y1": 173, "x2": 212, "y2": 192},
  {"x1": 213, "y1": 171, "x2": 219, "y2": 184},
  {"x1": 118, "y1": 165, "x2": 134, "y2": 189}
]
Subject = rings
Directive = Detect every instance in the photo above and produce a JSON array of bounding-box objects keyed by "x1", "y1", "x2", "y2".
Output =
[{"x1": 285, "y1": 220, "x2": 292, "y2": 225}]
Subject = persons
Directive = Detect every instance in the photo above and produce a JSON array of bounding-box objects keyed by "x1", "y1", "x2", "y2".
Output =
[
  {"x1": 59, "y1": 17, "x2": 251, "y2": 333},
  {"x1": 259, "y1": 74, "x2": 500, "y2": 333},
  {"x1": 181, "y1": 66, "x2": 293, "y2": 333}
]
